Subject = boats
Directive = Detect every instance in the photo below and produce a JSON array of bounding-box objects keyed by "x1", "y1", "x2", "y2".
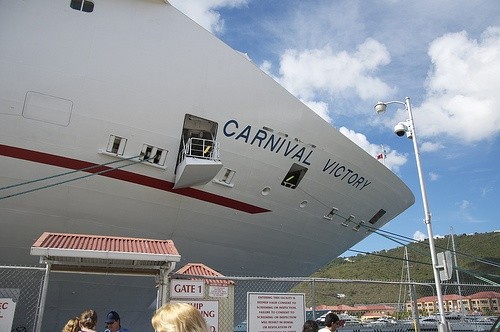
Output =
[{"x1": 0, "y1": 0, "x2": 416, "y2": 332}]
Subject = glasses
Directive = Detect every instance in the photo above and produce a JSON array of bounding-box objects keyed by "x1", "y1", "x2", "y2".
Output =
[
  {"x1": 333, "y1": 322, "x2": 339, "y2": 326},
  {"x1": 106, "y1": 321, "x2": 115, "y2": 325}
]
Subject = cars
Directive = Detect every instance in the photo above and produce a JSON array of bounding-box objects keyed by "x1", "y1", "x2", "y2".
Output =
[{"x1": 314, "y1": 311, "x2": 500, "y2": 326}]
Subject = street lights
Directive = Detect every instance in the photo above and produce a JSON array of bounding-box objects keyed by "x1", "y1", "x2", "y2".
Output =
[{"x1": 373, "y1": 96, "x2": 452, "y2": 332}]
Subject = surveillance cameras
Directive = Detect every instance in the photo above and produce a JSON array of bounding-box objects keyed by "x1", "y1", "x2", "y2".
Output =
[{"x1": 394, "y1": 124, "x2": 405, "y2": 136}]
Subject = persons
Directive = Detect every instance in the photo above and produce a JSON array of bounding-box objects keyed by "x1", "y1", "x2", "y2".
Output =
[
  {"x1": 317, "y1": 313, "x2": 340, "y2": 332},
  {"x1": 78, "y1": 309, "x2": 97, "y2": 332},
  {"x1": 62, "y1": 317, "x2": 81, "y2": 332},
  {"x1": 103, "y1": 311, "x2": 130, "y2": 332},
  {"x1": 151, "y1": 302, "x2": 210, "y2": 332},
  {"x1": 302, "y1": 319, "x2": 318, "y2": 332}
]
